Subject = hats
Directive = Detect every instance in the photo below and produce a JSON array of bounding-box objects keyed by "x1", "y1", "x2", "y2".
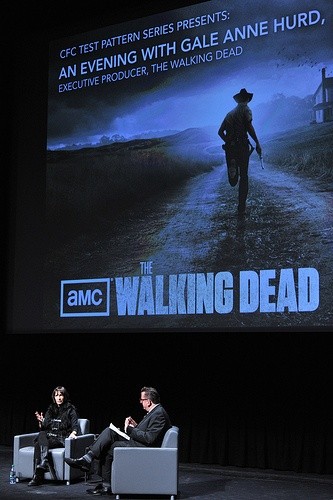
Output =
[{"x1": 233, "y1": 89, "x2": 253, "y2": 98}]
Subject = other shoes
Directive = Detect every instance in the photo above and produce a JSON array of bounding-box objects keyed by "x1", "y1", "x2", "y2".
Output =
[
  {"x1": 37, "y1": 458, "x2": 50, "y2": 472},
  {"x1": 229, "y1": 159, "x2": 236, "y2": 178},
  {"x1": 238, "y1": 205, "x2": 245, "y2": 210},
  {"x1": 27, "y1": 475, "x2": 44, "y2": 486}
]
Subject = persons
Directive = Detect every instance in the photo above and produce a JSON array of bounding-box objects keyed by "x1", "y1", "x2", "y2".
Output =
[
  {"x1": 27, "y1": 386, "x2": 79, "y2": 487},
  {"x1": 63, "y1": 387, "x2": 170, "y2": 495},
  {"x1": 218, "y1": 88, "x2": 262, "y2": 214}
]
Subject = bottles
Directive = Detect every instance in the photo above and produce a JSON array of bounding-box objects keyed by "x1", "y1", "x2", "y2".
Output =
[{"x1": 10, "y1": 465, "x2": 16, "y2": 484}]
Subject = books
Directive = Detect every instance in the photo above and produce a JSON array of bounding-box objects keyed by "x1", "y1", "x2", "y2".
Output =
[{"x1": 109, "y1": 423, "x2": 130, "y2": 440}]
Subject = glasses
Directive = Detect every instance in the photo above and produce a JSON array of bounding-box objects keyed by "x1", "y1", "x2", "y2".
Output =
[{"x1": 139, "y1": 398, "x2": 148, "y2": 401}]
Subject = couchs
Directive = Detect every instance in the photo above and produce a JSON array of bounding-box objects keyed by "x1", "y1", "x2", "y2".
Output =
[
  {"x1": 110, "y1": 426, "x2": 181, "y2": 500},
  {"x1": 14, "y1": 419, "x2": 94, "y2": 484}
]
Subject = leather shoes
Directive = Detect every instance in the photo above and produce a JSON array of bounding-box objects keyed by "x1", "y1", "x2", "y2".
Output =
[
  {"x1": 86, "y1": 484, "x2": 111, "y2": 496},
  {"x1": 64, "y1": 457, "x2": 91, "y2": 472}
]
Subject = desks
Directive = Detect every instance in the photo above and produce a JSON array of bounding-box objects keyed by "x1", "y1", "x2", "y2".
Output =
[{"x1": 84, "y1": 446, "x2": 104, "y2": 485}]
